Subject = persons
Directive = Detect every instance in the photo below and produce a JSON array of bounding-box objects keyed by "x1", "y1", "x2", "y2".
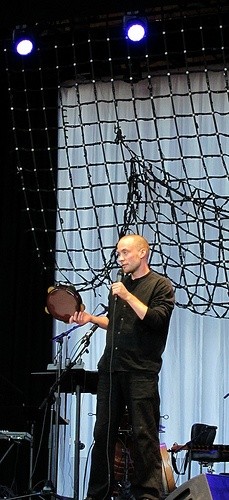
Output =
[{"x1": 67, "y1": 235, "x2": 176, "y2": 500}]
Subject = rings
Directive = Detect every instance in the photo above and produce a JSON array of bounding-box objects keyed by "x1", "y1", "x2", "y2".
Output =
[
  {"x1": 116, "y1": 289, "x2": 117, "y2": 291},
  {"x1": 77, "y1": 320, "x2": 83, "y2": 323}
]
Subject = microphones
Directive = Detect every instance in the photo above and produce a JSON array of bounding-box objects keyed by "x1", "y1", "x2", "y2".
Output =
[
  {"x1": 100, "y1": 303, "x2": 109, "y2": 311},
  {"x1": 114, "y1": 270, "x2": 124, "y2": 299},
  {"x1": 85, "y1": 324, "x2": 98, "y2": 339}
]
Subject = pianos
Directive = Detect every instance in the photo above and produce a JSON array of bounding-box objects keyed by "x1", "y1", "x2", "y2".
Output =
[{"x1": 31, "y1": 367, "x2": 98, "y2": 395}]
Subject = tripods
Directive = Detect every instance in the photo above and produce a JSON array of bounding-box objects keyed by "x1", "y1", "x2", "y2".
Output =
[{"x1": 0, "y1": 308, "x2": 106, "y2": 500}]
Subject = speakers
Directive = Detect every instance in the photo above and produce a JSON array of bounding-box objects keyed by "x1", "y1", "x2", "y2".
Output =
[{"x1": 164, "y1": 473, "x2": 229, "y2": 500}]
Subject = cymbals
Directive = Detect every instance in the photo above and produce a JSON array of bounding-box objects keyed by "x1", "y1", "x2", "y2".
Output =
[{"x1": 43, "y1": 284, "x2": 85, "y2": 324}]
